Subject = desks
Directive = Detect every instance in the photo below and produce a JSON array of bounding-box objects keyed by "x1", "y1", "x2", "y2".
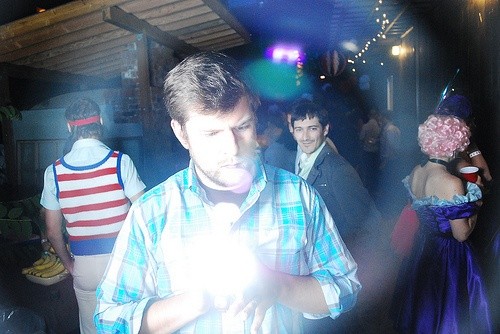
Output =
[{"x1": 0, "y1": 270, "x2": 81, "y2": 333}]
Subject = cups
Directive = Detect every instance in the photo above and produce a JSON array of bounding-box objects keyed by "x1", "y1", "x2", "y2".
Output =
[{"x1": 459, "y1": 166, "x2": 479, "y2": 183}]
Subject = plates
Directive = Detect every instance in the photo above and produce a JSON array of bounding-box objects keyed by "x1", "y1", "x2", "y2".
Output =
[{"x1": 25, "y1": 269, "x2": 70, "y2": 286}]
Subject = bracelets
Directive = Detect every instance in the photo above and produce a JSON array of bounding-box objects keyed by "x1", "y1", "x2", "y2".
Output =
[{"x1": 469, "y1": 150, "x2": 483, "y2": 158}]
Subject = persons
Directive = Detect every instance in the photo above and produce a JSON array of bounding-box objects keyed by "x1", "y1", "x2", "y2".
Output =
[
  {"x1": 253, "y1": 93, "x2": 500, "y2": 334},
  {"x1": 95, "y1": 52, "x2": 362, "y2": 334},
  {"x1": 41, "y1": 98, "x2": 146, "y2": 334}
]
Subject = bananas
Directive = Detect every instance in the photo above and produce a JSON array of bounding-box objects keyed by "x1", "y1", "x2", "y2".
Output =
[{"x1": 21, "y1": 247, "x2": 65, "y2": 278}]
